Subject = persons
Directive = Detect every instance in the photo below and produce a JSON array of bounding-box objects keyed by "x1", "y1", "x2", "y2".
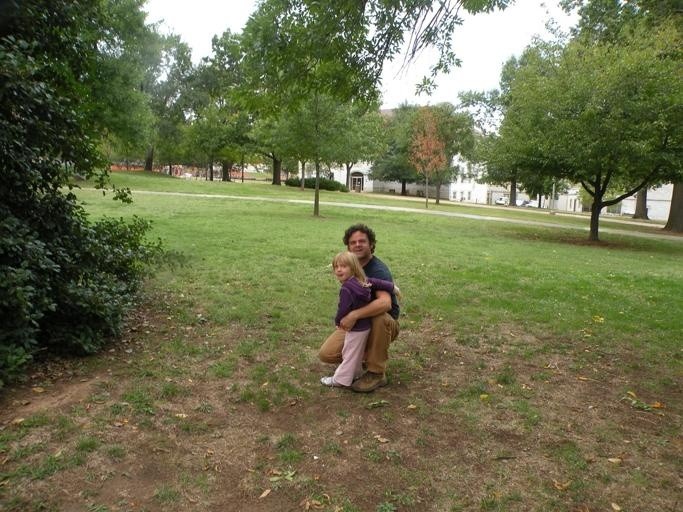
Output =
[
  {"x1": 319, "y1": 223, "x2": 400, "y2": 393},
  {"x1": 174, "y1": 167, "x2": 178, "y2": 176},
  {"x1": 320, "y1": 251, "x2": 403, "y2": 388}
]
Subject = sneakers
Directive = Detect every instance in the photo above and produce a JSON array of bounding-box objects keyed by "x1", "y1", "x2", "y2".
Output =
[
  {"x1": 352, "y1": 371, "x2": 387, "y2": 392},
  {"x1": 321, "y1": 377, "x2": 343, "y2": 386}
]
